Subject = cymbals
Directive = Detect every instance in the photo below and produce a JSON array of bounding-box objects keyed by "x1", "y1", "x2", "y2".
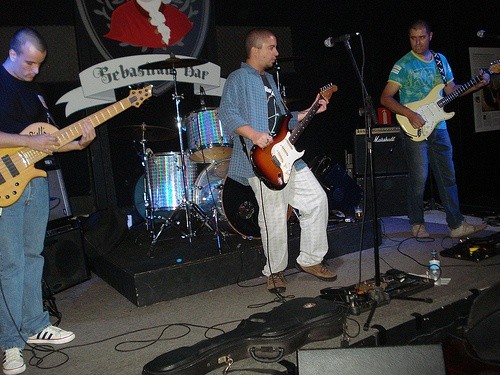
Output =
[
  {"x1": 281, "y1": 97, "x2": 303, "y2": 103},
  {"x1": 138, "y1": 58, "x2": 209, "y2": 70}
]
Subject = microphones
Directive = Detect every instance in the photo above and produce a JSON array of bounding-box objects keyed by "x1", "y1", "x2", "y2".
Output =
[{"x1": 323, "y1": 32, "x2": 360, "y2": 48}]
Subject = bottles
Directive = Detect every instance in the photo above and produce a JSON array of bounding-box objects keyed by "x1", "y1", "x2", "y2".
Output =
[{"x1": 428, "y1": 249, "x2": 441, "y2": 286}]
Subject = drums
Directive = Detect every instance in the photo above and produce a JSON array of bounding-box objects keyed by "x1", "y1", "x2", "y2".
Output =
[
  {"x1": 145, "y1": 152, "x2": 198, "y2": 211},
  {"x1": 184, "y1": 108, "x2": 233, "y2": 164},
  {"x1": 192, "y1": 157, "x2": 294, "y2": 242}
]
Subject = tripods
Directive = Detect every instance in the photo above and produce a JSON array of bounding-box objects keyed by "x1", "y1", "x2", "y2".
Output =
[
  {"x1": 331, "y1": 39, "x2": 435, "y2": 332},
  {"x1": 137, "y1": 65, "x2": 225, "y2": 245}
]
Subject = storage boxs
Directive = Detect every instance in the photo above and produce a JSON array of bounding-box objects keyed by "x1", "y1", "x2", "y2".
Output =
[
  {"x1": 354, "y1": 127, "x2": 415, "y2": 217},
  {"x1": 37, "y1": 155, "x2": 90, "y2": 298}
]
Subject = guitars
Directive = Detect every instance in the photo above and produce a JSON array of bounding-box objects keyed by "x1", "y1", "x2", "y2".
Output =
[
  {"x1": 0, "y1": 84, "x2": 153, "y2": 208},
  {"x1": 396, "y1": 60, "x2": 500, "y2": 142},
  {"x1": 250, "y1": 83, "x2": 338, "y2": 191}
]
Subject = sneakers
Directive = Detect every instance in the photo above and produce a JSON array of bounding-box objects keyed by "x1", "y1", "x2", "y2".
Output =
[
  {"x1": 411, "y1": 224, "x2": 429, "y2": 238},
  {"x1": 296, "y1": 260, "x2": 338, "y2": 282},
  {"x1": 266, "y1": 272, "x2": 286, "y2": 294},
  {"x1": 449, "y1": 222, "x2": 487, "y2": 239},
  {"x1": 3, "y1": 347, "x2": 26, "y2": 375},
  {"x1": 26, "y1": 324, "x2": 75, "y2": 344}
]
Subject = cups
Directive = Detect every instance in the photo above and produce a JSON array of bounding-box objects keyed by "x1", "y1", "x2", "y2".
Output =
[{"x1": 354, "y1": 206, "x2": 363, "y2": 218}]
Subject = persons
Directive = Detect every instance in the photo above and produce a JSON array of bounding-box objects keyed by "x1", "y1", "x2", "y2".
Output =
[
  {"x1": 380, "y1": 21, "x2": 490, "y2": 238},
  {"x1": 215, "y1": 29, "x2": 338, "y2": 293},
  {"x1": 0, "y1": 29, "x2": 97, "y2": 375}
]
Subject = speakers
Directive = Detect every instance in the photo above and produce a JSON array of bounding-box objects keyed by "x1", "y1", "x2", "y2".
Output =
[
  {"x1": 297, "y1": 341, "x2": 447, "y2": 375},
  {"x1": 353, "y1": 132, "x2": 413, "y2": 219},
  {"x1": 46, "y1": 167, "x2": 73, "y2": 221},
  {"x1": 465, "y1": 280, "x2": 500, "y2": 361},
  {"x1": 43, "y1": 227, "x2": 91, "y2": 300}
]
